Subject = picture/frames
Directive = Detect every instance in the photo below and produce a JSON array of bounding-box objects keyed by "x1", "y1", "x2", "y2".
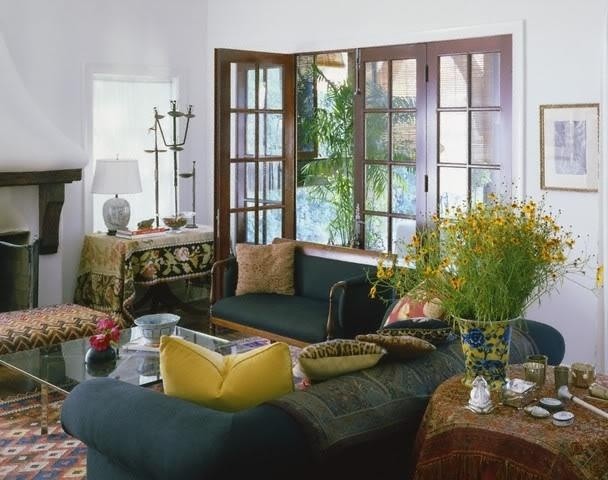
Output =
[{"x1": 536, "y1": 102, "x2": 600, "y2": 195}]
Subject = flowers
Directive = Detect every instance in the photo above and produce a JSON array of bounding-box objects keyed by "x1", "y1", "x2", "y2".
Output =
[
  {"x1": 89, "y1": 314, "x2": 123, "y2": 352},
  {"x1": 353, "y1": 164, "x2": 605, "y2": 336}
]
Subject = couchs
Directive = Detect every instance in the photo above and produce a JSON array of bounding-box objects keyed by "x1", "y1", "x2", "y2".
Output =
[
  {"x1": 207, "y1": 236, "x2": 397, "y2": 349},
  {"x1": 55, "y1": 319, "x2": 566, "y2": 478}
]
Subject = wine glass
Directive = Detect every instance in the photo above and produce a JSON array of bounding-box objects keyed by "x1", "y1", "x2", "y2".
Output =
[{"x1": 155, "y1": 211, "x2": 197, "y2": 234}]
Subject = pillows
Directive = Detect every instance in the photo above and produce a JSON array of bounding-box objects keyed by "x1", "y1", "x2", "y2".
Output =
[
  {"x1": 373, "y1": 319, "x2": 460, "y2": 346},
  {"x1": 354, "y1": 333, "x2": 438, "y2": 361},
  {"x1": 297, "y1": 338, "x2": 388, "y2": 381},
  {"x1": 157, "y1": 334, "x2": 295, "y2": 413},
  {"x1": 233, "y1": 242, "x2": 295, "y2": 297},
  {"x1": 380, "y1": 276, "x2": 451, "y2": 328}
]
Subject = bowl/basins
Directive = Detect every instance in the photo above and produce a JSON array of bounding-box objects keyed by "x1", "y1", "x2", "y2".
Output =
[{"x1": 132, "y1": 312, "x2": 182, "y2": 345}]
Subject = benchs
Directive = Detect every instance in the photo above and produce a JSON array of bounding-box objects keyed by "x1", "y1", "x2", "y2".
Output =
[{"x1": 0, "y1": 302, "x2": 117, "y2": 394}]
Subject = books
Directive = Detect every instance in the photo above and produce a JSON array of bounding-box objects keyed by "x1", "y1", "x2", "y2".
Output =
[{"x1": 122, "y1": 335, "x2": 185, "y2": 352}]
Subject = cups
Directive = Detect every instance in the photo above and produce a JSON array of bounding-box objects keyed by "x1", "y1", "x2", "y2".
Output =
[
  {"x1": 553, "y1": 364, "x2": 569, "y2": 393},
  {"x1": 572, "y1": 362, "x2": 597, "y2": 388},
  {"x1": 523, "y1": 354, "x2": 548, "y2": 391}
]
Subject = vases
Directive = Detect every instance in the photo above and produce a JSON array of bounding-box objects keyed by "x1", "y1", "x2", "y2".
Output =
[{"x1": 450, "y1": 314, "x2": 522, "y2": 391}]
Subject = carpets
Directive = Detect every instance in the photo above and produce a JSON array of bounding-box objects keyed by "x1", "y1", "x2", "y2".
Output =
[{"x1": 0, "y1": 332, "x2": 315, "y2": 479}]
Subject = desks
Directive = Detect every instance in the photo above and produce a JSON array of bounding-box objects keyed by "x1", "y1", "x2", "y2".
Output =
[
  {"x1": 73, "y1": 224, "x2": 216, "y2": 326},
  {"x1": 414, "y1": 363, "x2": 607, "y2": 478}
]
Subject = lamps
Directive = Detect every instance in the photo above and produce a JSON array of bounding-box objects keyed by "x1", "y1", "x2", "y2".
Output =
[{"x1": 89, "y1": 155, "x2": 142, "y2": 234}]
